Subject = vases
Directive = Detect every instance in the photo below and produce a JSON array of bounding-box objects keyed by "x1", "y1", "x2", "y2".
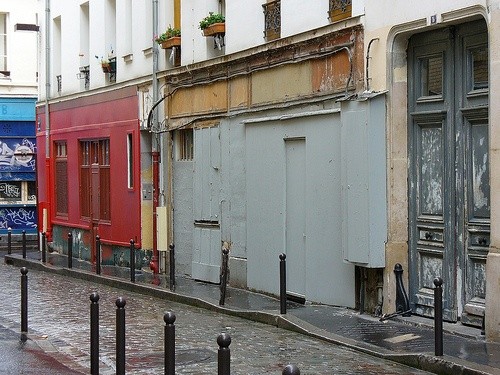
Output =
[{"x1": 160, "y1": 37, "x2": 181, "y2": 49}]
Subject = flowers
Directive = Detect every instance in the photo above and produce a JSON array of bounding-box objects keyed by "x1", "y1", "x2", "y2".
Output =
[{"x1": 153, "y1": 26, "x2": 181, "y2": 44}]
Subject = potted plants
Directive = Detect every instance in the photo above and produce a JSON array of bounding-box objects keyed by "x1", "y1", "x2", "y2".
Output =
[
  {"x1": 197, "y1": 11, "x2": 225, "y2": 36},
  {"x1": 95, "y1": 55, "x2": 116, "y2": 73}
]
